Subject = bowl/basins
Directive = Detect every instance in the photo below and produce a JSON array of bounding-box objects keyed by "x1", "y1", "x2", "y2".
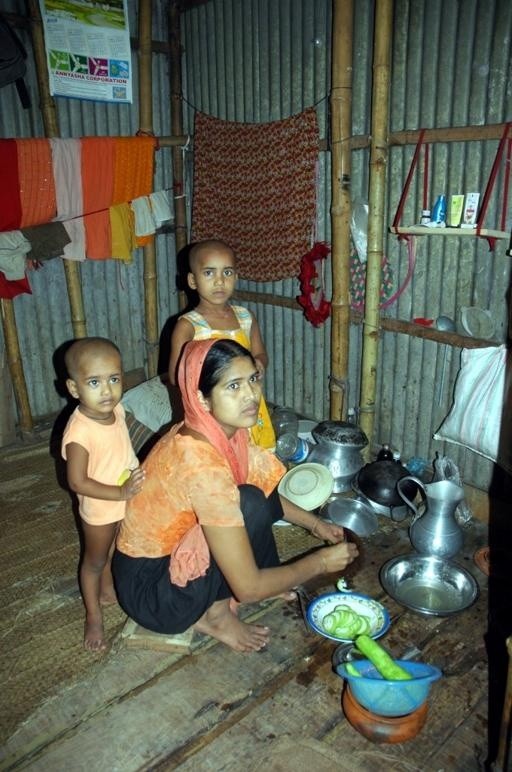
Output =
[
  {"x1": 335, "y1": 662, "x2": 441, "y2": 715},
  {"x1": 297, "y1": 420, "x2": 317, "y2": 440}
]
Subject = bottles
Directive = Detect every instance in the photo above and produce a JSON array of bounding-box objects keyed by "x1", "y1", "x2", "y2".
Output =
[
  {"x1": 377, "y1": 443, "x2": 393, "y2": 461},
  {"x1": 276, "y1": 433, "x2": 315, "y2": 465},
  {"x1": 271, "y1": 408, "x2": 298, "y2": 435},
  {"x1": 420, "y1": 211, "x2": 431, "y2": 226}
]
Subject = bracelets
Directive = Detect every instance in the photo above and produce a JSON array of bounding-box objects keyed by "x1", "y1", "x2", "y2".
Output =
[
  {"x1": 310, "y1": 516, "x2": 321, "y2": 537},
  {"x1": 313, "y1": 550, "x2": 328, "y2": 575}
]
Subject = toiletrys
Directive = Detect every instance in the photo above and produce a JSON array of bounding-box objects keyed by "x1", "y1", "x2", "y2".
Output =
[
  {"x1": 463, "y1": 192, "x2": 481, "y2": 226},
  {"x1": 449, "y1": 195, "x2": 464, "y2": 227},
  {"x1": 434, "y1": 196, "x2": 446, "y2": 224}
]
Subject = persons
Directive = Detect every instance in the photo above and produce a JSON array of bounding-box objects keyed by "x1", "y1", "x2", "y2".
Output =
[
  {"x1": 168, "y1": 239, "x2": 276, "y2": 455},
  {"x1": 111, "y1": 338, "x2": 360, "y2": 654},
  {"x1": 60, "y1": 336, "x2": 147, "y2": 652}
]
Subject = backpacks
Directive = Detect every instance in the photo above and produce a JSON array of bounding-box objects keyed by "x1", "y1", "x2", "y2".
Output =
[{"x1": 0, "y1": 19, "x2": 28, "y2": 88}]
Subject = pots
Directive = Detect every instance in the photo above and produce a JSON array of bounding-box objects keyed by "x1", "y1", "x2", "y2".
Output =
[{"x1": 358, "y1": 461, "x2": 419, "y2": 522}]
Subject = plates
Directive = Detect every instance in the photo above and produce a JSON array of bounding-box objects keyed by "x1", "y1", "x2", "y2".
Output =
[
  {"x1": 376, "y1": 554, "x2": 479, "y2": 615},
  {"x1": 308, "y1": 593, "x2": 390, "y2": 644},
  {"x1": 277, "y1": 461, "x2": 333, "y2": 511}
]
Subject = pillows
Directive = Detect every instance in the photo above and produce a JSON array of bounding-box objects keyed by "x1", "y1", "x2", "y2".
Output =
[{"x1": 120, "y1": 376, "x2": 174, "y2": 433}]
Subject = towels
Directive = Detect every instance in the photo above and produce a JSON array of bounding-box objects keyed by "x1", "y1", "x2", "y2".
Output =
[
  {"x1": 191, "y1": 107, "x2": 317, "y2": 282},
  {"x1": 0, "y1": 136, "x2": 174, "y2": 299}
]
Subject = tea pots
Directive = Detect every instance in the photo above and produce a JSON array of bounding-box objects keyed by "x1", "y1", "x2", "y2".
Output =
[{"x1": 397, "y1": 476, "x2": 465, "y2": 557}]
